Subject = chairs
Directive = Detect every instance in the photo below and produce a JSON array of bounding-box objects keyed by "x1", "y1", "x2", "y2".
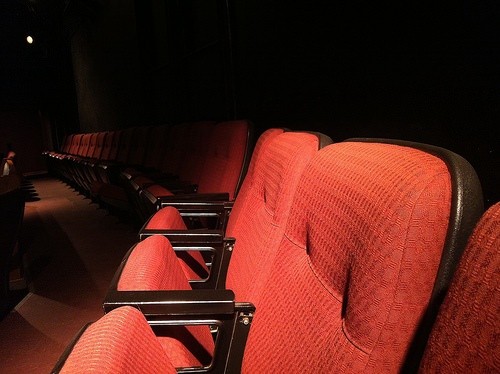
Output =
[
  {"x1": 137, "y1": 127, "x2": 288, "y2": 295},
  {"x1": 51, "y1": 137, "x2": 488, "y2": 374},
  {"x1": 41, "y1": 112, "x2": 256, "y2": 221},
  {"x1": 105, "y1": 126, "x2": 329, "y2": 374}
]
